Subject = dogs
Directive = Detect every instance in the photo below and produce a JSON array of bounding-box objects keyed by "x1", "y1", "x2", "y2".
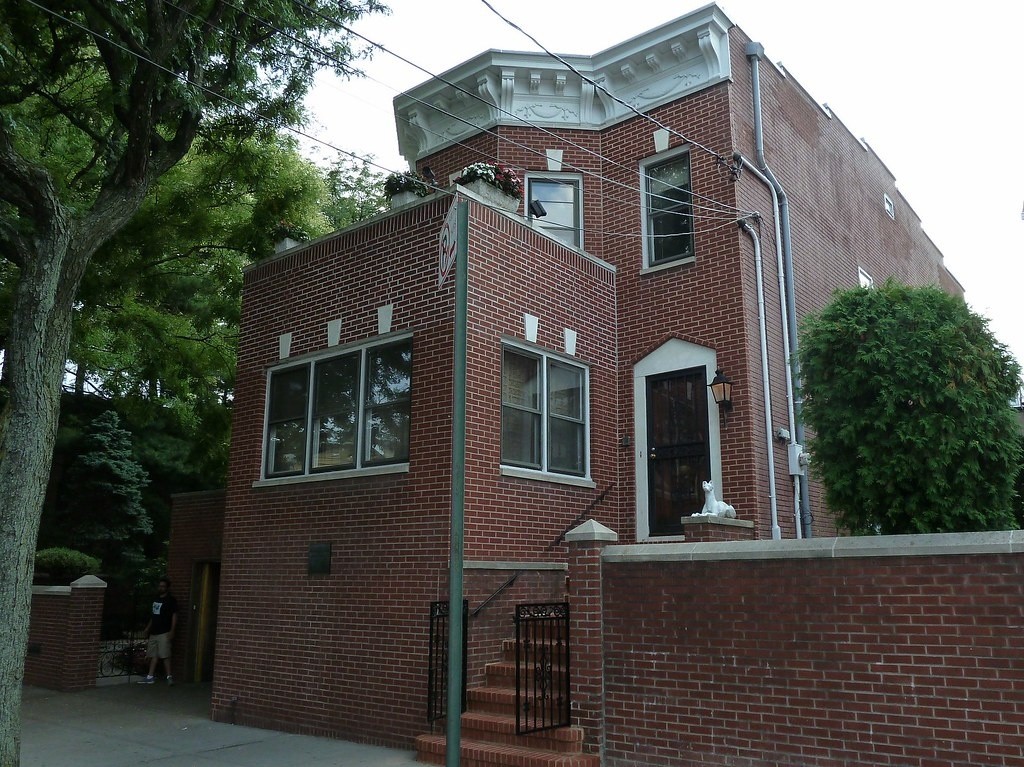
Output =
[{"x1": 690, "y1": 479, "x2": 737, "y2": 519}]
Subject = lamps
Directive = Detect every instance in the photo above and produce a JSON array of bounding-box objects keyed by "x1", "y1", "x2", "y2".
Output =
[
  {"x1": 707, "y1": 370, "x2": 734, "y2": 410},
  {"x1": 528, "y1": 199, "x2": 546, "y2": 219}
]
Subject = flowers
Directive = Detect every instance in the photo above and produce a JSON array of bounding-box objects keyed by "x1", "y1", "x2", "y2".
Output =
[
  {"x1": 452, "y1": 162, "x2": 523, "y2": 201},
  {"x1": 382, "y1": 169, "x2": 431, "y2": 202}
]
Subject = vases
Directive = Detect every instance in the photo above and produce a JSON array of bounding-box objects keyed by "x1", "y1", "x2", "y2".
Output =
[
  {"x1": 391, "y1": 191, "x2": 420, "y2": 210},
  {"x1": 462, "y1": 178, "x2": 520, "y2": 212}
]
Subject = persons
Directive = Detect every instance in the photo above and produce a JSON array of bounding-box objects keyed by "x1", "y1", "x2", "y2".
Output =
[{"x1": 137, "y1": 578, "x2": 177, "y2": 686}]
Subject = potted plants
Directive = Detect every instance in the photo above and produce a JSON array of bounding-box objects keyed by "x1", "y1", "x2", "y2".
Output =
[{"x1": 267, "y1": 220, "x2": 311, "y2": 254}]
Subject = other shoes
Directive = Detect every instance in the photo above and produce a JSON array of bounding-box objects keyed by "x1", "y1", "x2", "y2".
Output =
[
  {"x1": 165, "y1": 671, "x2": 177, "y2": 686},
  {"x1": 137, "y1": 674, "x2": 156, "y2": 684}
]
